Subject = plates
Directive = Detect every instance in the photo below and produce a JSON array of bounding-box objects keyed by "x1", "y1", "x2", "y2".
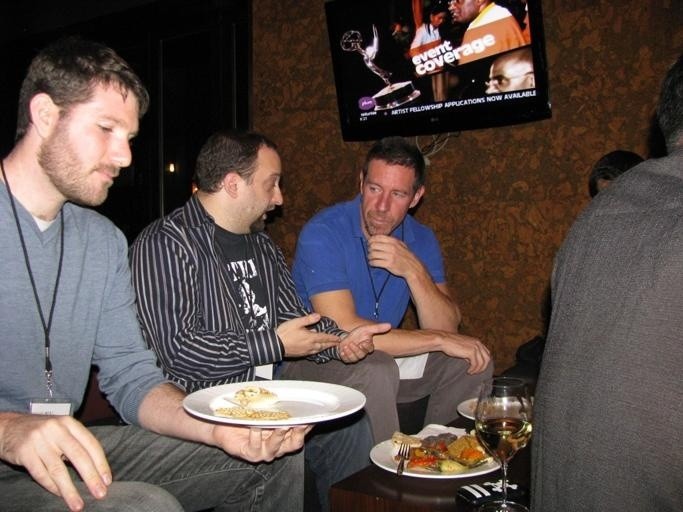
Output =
[
  {"x1": 181, "y1": 379, "x2": 367, "y2": 428},
  {"x1": 368, "y1": 433, "x2": 502, "y2": 480},
  {"x1": 457, "y1": 397, "x2": 532, "y2": 420}
]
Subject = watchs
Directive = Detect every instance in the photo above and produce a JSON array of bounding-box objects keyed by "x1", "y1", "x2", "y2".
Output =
[{"x1": 278, "y1": 336, "x2": 285, "y2": 357}]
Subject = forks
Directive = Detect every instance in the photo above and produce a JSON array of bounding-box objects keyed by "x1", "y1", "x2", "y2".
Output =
[{"x1": 394, "y1": 441, "x2": 411, "y2": 479}]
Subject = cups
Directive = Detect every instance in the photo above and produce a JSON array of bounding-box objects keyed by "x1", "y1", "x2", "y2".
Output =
[{"x1": 474, "y1": 376, "x2": 533, "y2": 512}]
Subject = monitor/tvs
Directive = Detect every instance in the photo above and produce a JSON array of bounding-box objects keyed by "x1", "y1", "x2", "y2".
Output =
[{"x1": 324, "y1": 0, "x2": 552, "y2": 144}]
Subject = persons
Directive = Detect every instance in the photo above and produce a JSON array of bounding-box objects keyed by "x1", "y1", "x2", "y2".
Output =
[
  {"x1": 407, "y1": 2, "x2": 459, "y2": 104},
  {"x1": 126, "y1": 129, "x2": 402, "y2": 451},
  {"x1": 530, "y1": 51, "x2": 681, "y2": 512},
  {"x1": 0, "y1": 37, "x2": 309, "y2": 512},
  {"x1": 288, "y1": 140, "x2": 498, "y2": 452},
  {"x1": 588, "y1": 150, "x2": 645, "y2": 199},
  {"x1": 447, "y1": 0, "x2": 525, "y2": 66},
  {"x1": 483, "y1": 47, "x2": 537, "y2": 95}
]
